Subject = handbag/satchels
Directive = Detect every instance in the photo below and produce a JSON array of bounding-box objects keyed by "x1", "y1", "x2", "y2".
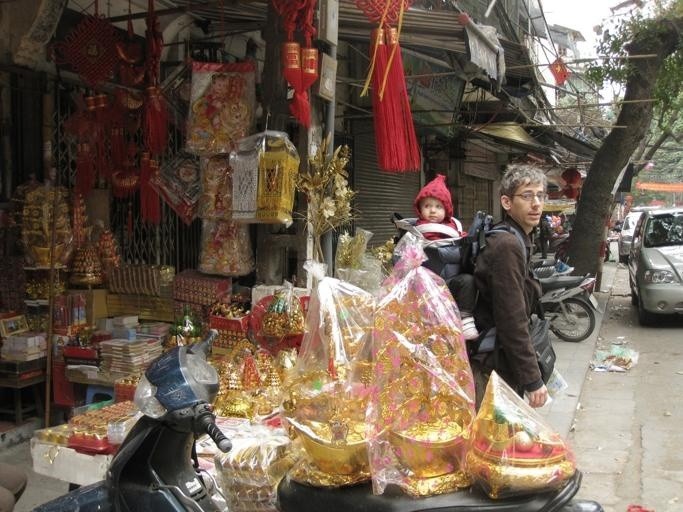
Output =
[{"x1": 487, "y1": 318, "x2": 556, "y2": 384}]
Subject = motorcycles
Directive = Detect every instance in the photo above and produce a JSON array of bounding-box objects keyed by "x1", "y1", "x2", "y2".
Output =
[
  {"x1": 528, "y1": 257, "x2": 575, "y2": 278},
  {"x1": 26, "y1": 325, "x2": 606, "y2": 512},
  {"x1": 531, "y1": 276, "x2": 599, "y2": 346}
]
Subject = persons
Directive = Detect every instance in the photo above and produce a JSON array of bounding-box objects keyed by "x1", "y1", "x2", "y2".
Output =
[
  {"x1": 411, "y1": 172, "x2": 480, "y2": 342},
  {"x1": 204, "y1": 73, "x2": 231, "y2": 132},
  {"x1": 467, "y1": 162, "x2": 557, "y2": 419},
  {"x1": 537, "y1": 214, "x2": 550, "y2": 261}
]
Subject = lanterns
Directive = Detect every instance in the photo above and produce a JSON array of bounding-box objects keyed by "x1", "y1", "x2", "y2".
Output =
[
  {"x1": 561, "y1": 168, "x2": 581, "y2": 184},
  {"x1": 562, "y1": 187, "x2": 578, "y2": 199}
]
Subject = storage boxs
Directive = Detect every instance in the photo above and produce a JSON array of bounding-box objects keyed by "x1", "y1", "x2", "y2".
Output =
[
  {"x1": 28, "y1": 439, "x2": 114, "y2": 487},
  {"x1": 65, "y1": 288, "x2": 107, "y2": 319}
]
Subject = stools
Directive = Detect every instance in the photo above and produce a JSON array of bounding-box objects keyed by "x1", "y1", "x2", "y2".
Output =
[
  {"x1": 82, "y1": 382, "x2": 116, "y2": 406},
  {"x1": 0, "y1": 372, "x2": 45, "y2": 427}
]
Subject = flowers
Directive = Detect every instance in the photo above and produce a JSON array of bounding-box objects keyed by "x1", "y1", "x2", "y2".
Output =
[{"x1": 283, "y1": 128, "x2": 360, "y2": 264}]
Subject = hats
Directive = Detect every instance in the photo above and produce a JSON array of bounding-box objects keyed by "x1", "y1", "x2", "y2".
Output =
[{"x1": 414, "y1": 175, "x2": 453, "y2": 219}]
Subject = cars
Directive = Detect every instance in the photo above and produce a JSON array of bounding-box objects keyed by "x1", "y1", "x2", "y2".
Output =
[
  {"x1": 626, "y1": 206, "x2": 683, "y2": 325},
  {"x1": 618, "y1": 205, "x2": 661, "y2": 261}
]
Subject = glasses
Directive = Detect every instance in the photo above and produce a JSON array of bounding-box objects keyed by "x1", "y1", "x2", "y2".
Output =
[{"x1": 512, "y1": 193, "x2": 544, "y2": 200}]
